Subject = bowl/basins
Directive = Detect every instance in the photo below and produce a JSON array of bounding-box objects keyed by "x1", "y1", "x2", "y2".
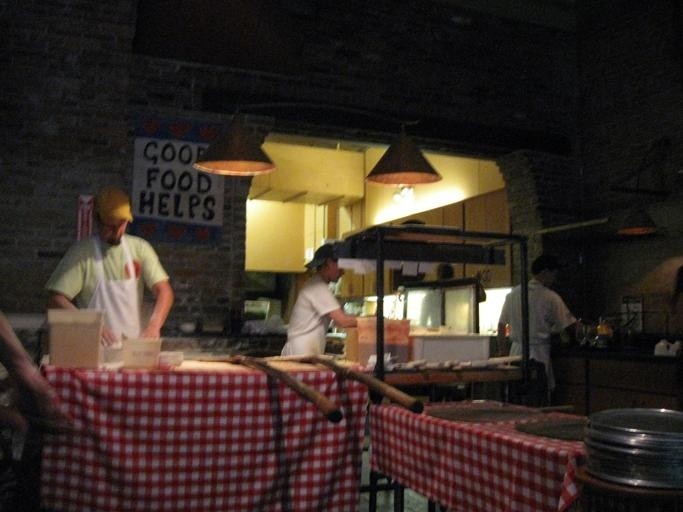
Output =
[{"x1": 158, "y1": 350, "x2": 182, "y2": 371}]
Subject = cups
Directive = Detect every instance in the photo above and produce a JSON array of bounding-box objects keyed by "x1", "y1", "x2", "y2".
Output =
[{"x1": 596, "y1": 324, "x2": 610, "y2": 347}]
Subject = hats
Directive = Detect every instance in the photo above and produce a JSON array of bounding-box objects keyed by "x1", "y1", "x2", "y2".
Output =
[
  {"x1": 304, "y1": 245, "x2": 338, "y2": 269},
  {"x1": 95, "y1": 186, "x2": 133, "y2": 224}
]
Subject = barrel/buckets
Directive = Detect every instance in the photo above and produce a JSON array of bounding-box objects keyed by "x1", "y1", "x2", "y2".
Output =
[{"x1": 358, "y1": 317, "x2": 410, "y2": 364}]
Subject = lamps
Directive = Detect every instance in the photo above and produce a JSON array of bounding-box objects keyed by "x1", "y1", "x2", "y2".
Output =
[
  {"x1": 364, "y1": 127, "x2": 443, "y2": 184},
  {"x1": 192, "y1": 107, "x2": 277, "y2": 176}
]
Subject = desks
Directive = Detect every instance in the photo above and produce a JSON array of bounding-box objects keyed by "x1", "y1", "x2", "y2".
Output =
[
  {"x1": 368, "y1": 400, "x2": 593, "y2": 512},
  {"x1": 359, "y1": 357, "x2": 545, "y2": 418},
  {"x1": 35, "y1": 351, "x2": 369, "y2": 512}
]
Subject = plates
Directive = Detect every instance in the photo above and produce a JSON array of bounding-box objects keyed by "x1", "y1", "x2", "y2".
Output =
[{"x1": 581, "y1": 406, "x2": 681, "y2": 489}]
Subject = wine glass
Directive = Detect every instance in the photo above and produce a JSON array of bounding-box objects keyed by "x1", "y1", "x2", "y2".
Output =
[{"x1": 574, "y1": 323, "x2": 598, "y2": 344}]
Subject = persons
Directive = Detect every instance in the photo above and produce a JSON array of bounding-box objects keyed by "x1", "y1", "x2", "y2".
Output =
[
  {"x1": 43, "y1": 184, "x2": 175, "y2": 347},
  {"x1": 495, "y1": 255, "x2": 578, "y2": 406},
  {"x1": 419, "y1": 263, "x2": 486, "y2": 401},
  {"x1": 280, "y1": 242, "x2": 358, "y2": 358}
]
